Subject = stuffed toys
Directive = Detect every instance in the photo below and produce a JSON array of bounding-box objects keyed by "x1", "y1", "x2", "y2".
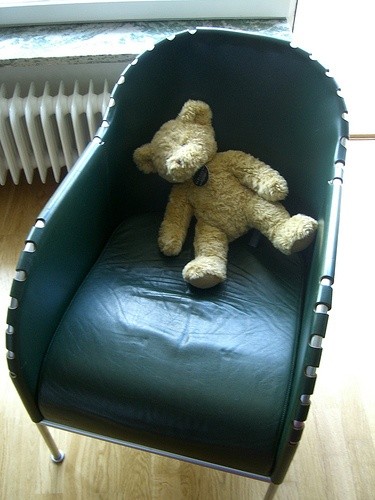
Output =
[{"x1": 133, "y1": 99, "x2": 318, "y2": 289}]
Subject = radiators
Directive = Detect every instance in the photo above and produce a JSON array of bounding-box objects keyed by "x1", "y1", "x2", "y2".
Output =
[{"x1": 0, "y1": 78, "x2": 112, "y2": 186}]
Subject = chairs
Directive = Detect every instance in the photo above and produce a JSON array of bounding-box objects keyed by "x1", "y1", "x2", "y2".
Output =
[{"x1": 4, "y1": 25, "x2": 351, "y2": 500}]
